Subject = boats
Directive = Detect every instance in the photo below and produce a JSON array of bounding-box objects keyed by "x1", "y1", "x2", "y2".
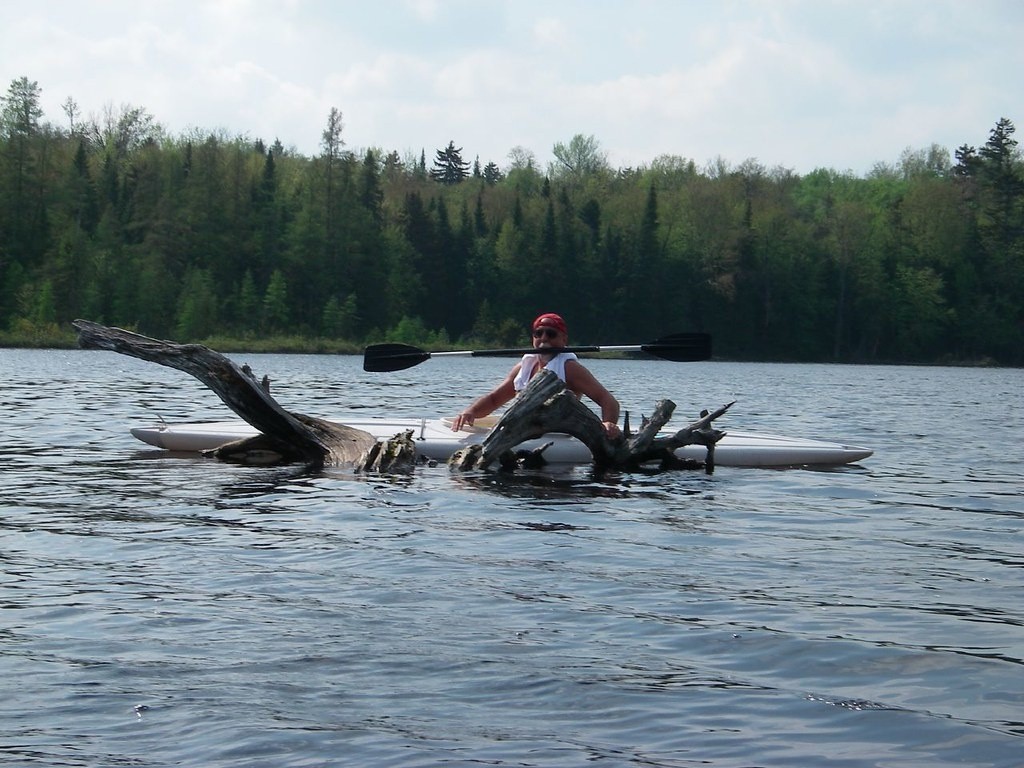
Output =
[{"x1": 128, "y1": 416, "x2": 877, "y2": 475}]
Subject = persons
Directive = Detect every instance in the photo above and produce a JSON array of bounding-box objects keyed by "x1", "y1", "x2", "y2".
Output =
[{"x1": 452, "y1": 314, "x2": 621, "y2": 439}]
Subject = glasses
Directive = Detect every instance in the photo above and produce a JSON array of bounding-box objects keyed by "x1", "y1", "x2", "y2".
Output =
[
  {"x1": 532, "y1": 327, "x2": 558, "y2": 340},
  {"x1": 537, "y1": 317, "x2": 556, "y2": 326}
]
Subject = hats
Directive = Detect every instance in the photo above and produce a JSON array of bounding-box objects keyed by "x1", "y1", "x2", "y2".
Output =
[{"x1": 532, "y1": 314, "x2": 569, "y2": 334}]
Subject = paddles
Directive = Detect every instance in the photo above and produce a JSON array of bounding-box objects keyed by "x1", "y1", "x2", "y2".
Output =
[{"x1": 363, "y1": 331, "x2": 713, "y2": 374}]
